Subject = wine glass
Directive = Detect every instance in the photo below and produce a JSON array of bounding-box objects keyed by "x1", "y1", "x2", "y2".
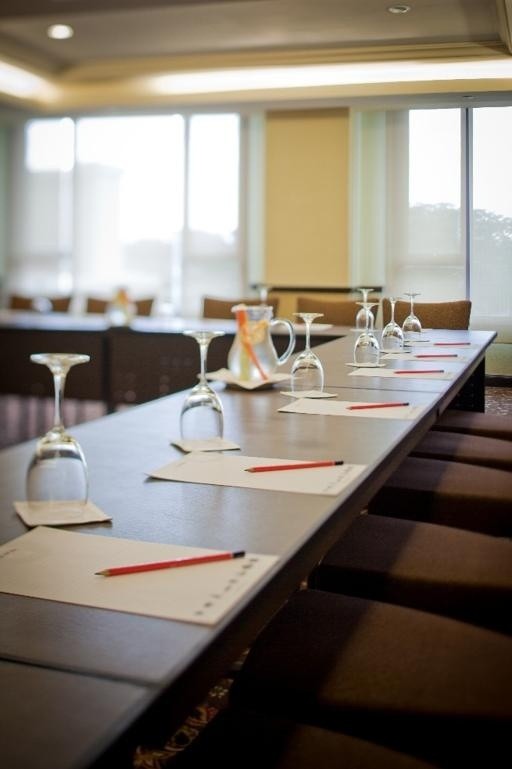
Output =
[
  {"x1": 355, "y1": 288, "x2": 376, "y2": 332},
  {"x1": 403, "y1": 292, "x2": 423, "y2": 340},
  {"x1": 383, "y1": 296, "x2": 405, "y2": 351},
  {"x1": 291, "y1": 310, "x2": 323, "y2": 394},
  {"x1": 22, "y1": 353, "x2": 92, "y2": 507},
  {"x1": 179, "y1": 328, "x2": 223, "y2": 440},
  {"x1": 354, "y1": 301, "x2": 380, "y2": 364}
]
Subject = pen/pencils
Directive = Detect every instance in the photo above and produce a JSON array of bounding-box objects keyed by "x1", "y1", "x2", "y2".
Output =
[
  {"x1": 433, "y1": 343, "x2": 470, "y2": 345},
  {"x1": 394, "y1": 370, "x2": 444, "y2": 373},
  {"x1": 344, "y1": 402, "x2": 409, "y2": 410},
  {"x1": 414, "y1": 355, "x2": 457, "y2": 357},
  {"x1": 244, "y1": 460, "x2": 344, "y2": 472},
  {"x1": 93, "y1": 550, "x2": 246, "y2": 577}
]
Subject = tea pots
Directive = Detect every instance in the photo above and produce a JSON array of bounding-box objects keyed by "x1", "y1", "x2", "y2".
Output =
[{"x1": 226, "y1": 305, "x2": 298, "y2": 383}]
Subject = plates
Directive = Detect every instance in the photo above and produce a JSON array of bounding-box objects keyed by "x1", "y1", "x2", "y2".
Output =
[{"x1": 195, "y1": 367, "x2": 294, "y2": 391}]
